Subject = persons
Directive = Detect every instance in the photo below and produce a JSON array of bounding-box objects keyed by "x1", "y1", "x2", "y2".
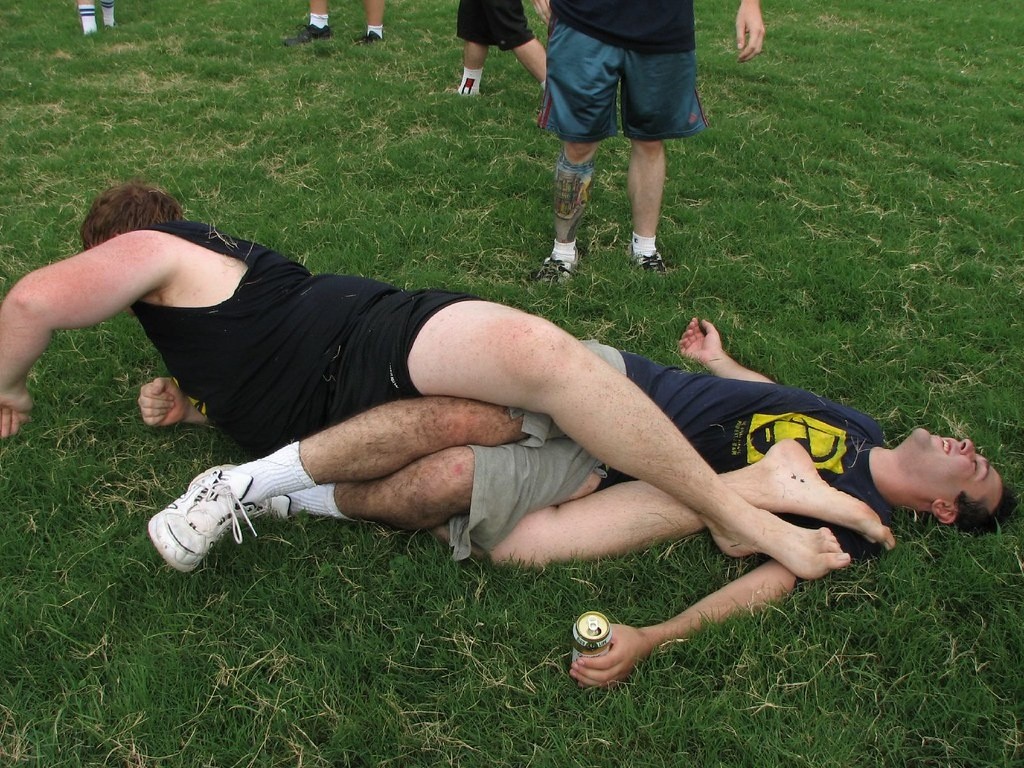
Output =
[
  {"x1": 284, "y1": 0, "x2": 385, "y2": 44},
  {"x1": 76, "y1": 0, "x2": 117, "y2": 35},
  {"x1": 531, "y1": 0, "x2": 710, "y2": 288},
  {"x1": 0, "y1": 181, "x2": 895, "y2": 582},
  {"x1": 439, "y1": 0, "x2": 548, "y2": 95},
  {"x1": 149, "y1": 316, "x2": 1015, "y2": 690}
]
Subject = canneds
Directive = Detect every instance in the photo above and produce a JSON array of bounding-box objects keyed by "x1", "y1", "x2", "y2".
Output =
[{"x1": 571, "y1": 611, "x2": 612, "y2": 662}]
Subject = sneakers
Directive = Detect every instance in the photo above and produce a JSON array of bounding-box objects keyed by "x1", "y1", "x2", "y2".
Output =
[
  {"x1": 626, "y1": 243, "x2": 668, "y2": 278},
  {"x1": 188, "y1": 464, "x2": 291, "y2": 522},
  {"x1": 355, "y1": 31, "x2": 383, "y2": 46},
  {"x1": 535, "y1": 246, "x2": 578, "y2": 287},
  {"x1": 284, "y1": 24, "x2": 330, "y2": 46},
  {"x1": 148, "y1": 470, "x2": 257, "y2": 573}
]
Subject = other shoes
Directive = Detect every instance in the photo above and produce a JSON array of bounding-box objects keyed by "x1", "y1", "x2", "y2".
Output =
[{"x1": 104, "y1": 21, "x2": 118, "y2": 31}]
